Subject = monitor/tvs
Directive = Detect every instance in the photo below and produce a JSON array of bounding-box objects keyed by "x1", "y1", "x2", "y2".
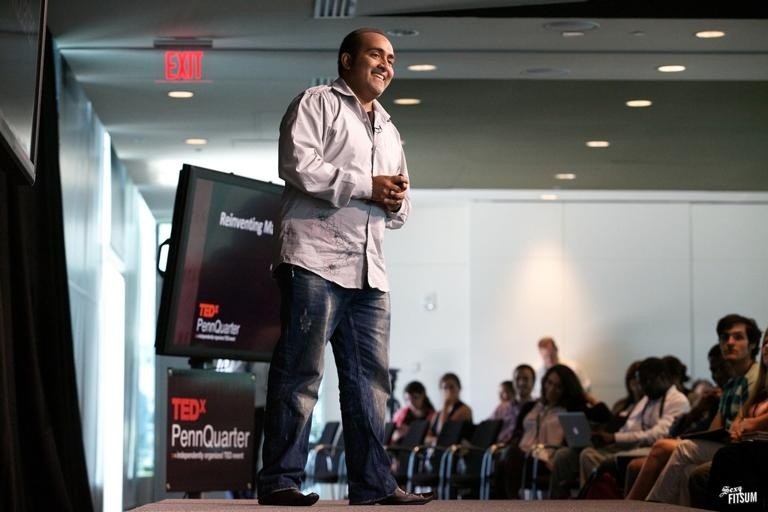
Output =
[
  {"x1": 154, "y1": 164, "x2": 285, "y2": 361},
  {"x1": 0, "y1": 0, "x2": 48, "y2": 187}
]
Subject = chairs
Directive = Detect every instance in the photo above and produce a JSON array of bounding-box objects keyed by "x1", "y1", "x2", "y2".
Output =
[{"x1": 301, "y1": 395, "x2": 722, "y2": 506}]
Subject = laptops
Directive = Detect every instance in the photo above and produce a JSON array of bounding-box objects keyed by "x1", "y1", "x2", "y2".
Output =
[{"x1": 557, "y1": 413, "x2": 623, "y2": 450}]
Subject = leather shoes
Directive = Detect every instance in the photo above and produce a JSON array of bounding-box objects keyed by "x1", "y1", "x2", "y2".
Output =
[
  {"x1": 349, "y1": 485, "x2": 435, "y2": 506},
  {"x1": 258, "y1": 486, "x2": 319, "y2": 506}
]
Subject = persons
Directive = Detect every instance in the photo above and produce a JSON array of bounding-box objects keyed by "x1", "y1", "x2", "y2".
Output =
[
  {"x1": 390, "y1": 310, "x2": 766, "y2": 510},
  {"x1": 254, "y1": 26, "x2": 436, "y2": 504}
]
